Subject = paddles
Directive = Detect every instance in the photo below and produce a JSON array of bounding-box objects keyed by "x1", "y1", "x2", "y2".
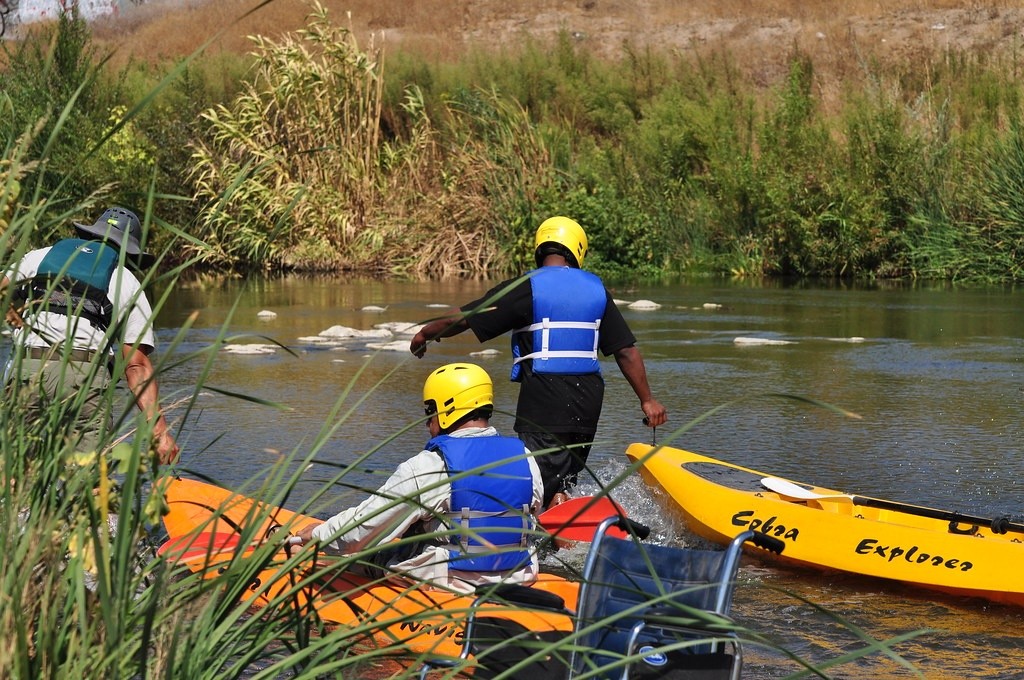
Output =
[
  {"x1": 759, "y1": 476, "x2": 1024, "y2": 536},
  {"x1": 155, "y1": 492, "x2": 630, "y2": 569}
]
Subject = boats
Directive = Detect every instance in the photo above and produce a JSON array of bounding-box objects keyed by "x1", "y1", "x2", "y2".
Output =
[
  {"x1": 620, "y1": 439, "x2": 1024, "y2": 607},
  {"x1": 142, "y1": 476, "x2": 581, "y2": 666}
]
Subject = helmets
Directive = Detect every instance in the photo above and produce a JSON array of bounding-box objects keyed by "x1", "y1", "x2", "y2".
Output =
[
  {"x1": 535, "y1": 216, "x2": 589, "y2": 270},
  {"x1": 423, "y1": 362, "x2": 494, "y2": 430}
]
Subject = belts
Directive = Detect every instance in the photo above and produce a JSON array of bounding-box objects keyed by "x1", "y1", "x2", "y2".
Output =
[
  {"x1": 449, "y1": 566, "x2": 527, "y2": 579},
  {"x1": 22, "y1": 347, "x2": 105, "y2": 366}
]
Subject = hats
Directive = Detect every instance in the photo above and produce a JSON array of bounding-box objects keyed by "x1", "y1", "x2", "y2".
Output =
[{"x1": 71, "y1": 207, "x2": 155, "y2": 271}]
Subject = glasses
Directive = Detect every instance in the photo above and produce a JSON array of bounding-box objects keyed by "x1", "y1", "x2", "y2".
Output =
[{"x1": 424, "y1": 403, "x2": 437, "y2": 422}]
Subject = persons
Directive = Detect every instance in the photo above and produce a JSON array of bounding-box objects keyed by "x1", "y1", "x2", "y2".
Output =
[
  {"x1": 0, "y1": 206, "x2": 181, "y2": 469},
  {"x1": 296, "y1": 361, "x2": 545, "y2": 597},
  {"x1": 410, "y1": 216, "x2": 668, "y2": 510}
]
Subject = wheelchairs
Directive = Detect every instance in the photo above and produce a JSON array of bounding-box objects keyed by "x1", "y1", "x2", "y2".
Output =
[{"x1": 416, "y1": 513, "x2": 787, "y2": 680}]
